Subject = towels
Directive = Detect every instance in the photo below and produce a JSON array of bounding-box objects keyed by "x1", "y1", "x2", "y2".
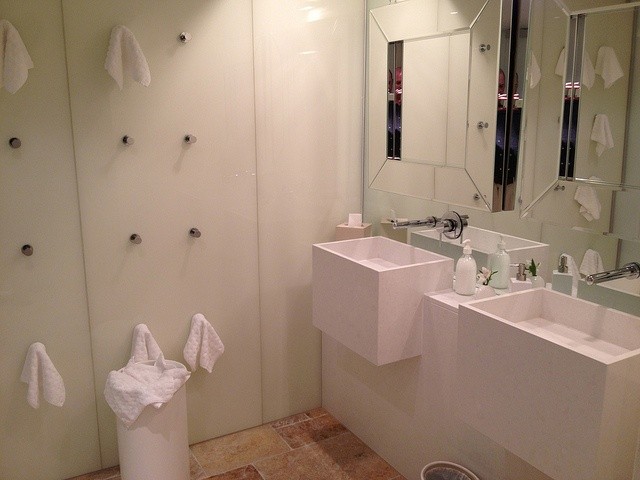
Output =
[
  {"x1": 183, "y1": 313, "x2": 224, "y2": 374},
  {"x1": 574, "y1": 185, "x2": 603, "y2": 222},
  {"x1": 594, "y1": 46, "x2": 625, "y2": 89},
  {"x1": 131, "y1": 324, "x2": 165, "y2": 361},
  {"x1": 582, "y1": 48, "x2": 595, "y2": 91},
  {"x1": 20, "y1": 342, "x2": 66, "y2": 410},
  {"x1": 104, "y1": 25, "x2": 151, "y2": 90},
  {"x1": 0, "y1": 19, "x2": 34, "y2": 95},
  {"x1": 555, "y1": 47, "x2": 566, "y2": 77},
  {"x1": 526, "y1": 50, "x2": 541, "y2": 89},
  {"x1": 591, "y1": 114, "x2": 614, "y2": 158},
  {"x1": 104, "y1": 354, "x2": 191, "y2": 429}
]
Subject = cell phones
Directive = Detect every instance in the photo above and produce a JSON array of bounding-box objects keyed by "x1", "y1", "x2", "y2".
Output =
[
  {"x1": 565, "y1": 86, "x2": 572, "y2": 98},
  {"x1": 388, "y1": 93, "x2": 394, "y2": 101},
  {"x1": 574, "y1": 86, "x2": 581, "y2": 97},
  {"x1": 397, "y1": 90, "x2": 402, "y2": 95},
  {"x1": 498, "y1": 96, "x2": 507, "y2": 108},
  {"x1": 515, "y1": 99, "x2": 523, "y2": 108}
]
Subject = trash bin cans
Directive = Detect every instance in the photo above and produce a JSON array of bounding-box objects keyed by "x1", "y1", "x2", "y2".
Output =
[{"x1": 420, "y1": 461, "x2": 479, "y2": 480}]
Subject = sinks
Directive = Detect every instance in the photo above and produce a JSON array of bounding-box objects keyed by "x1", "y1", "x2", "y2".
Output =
[
  {"x1": 469, "y1": 289, "x2": 640, "y2": 360},
  {"x1": 317, "y1": 237, "x2": 448, "y2": 270}
]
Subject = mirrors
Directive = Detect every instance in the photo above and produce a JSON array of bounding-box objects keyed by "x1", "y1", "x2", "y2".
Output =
[{"x1": 515, "y1": 0, "x2": 640, "y2": 243}]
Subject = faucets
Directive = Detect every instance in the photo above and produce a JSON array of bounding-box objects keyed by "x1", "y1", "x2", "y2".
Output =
[
  {"x1": 392, "y1": 217, "x2": 435, "y2": 230},
  {"x1": 586, "y1": 264, "x2": 639, "y2": 288}
]
